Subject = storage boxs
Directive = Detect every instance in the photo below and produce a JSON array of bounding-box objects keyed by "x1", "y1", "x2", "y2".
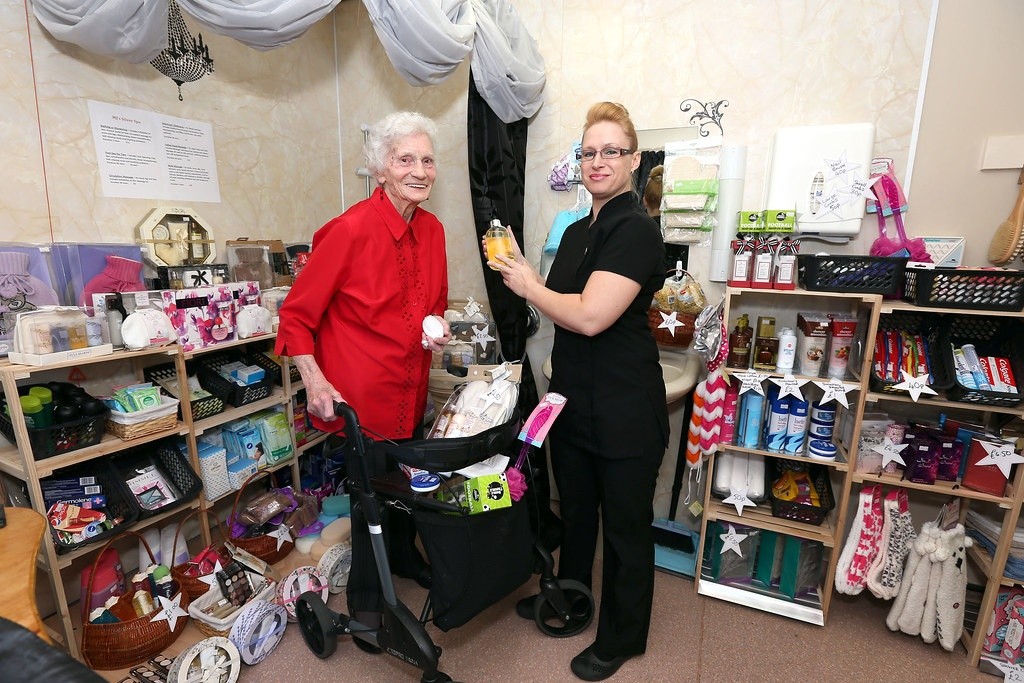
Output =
[
  {"x1": 867, "y1": 309, "x2": 957, "y2": 398},
  {"x1": 143, "y1": 359, "x2": 234, "y2": 422},
  {"x1": 943, "y1": 314, "x2": 1024, "y2": 408},
  {"x1": 901, "y1": 266, "x2": 1024, "y2": 309},
  {"x1": 194, "y1": 348, "x2": 274, "y2": 407},
  {"x1": 107, "y1": 436, "x2": 204, "y2": 520},
  {"x1": 17, "y1": 457, "x2": 142, "y2": 555},
  {"x1": 764, "y1": 454, "x2": 836, "y2": 527},
  {"x1": 796, "y1": 251, "x2": 909, "y2": 293},
  {"x1": 245, "y1": 339, "x2": 302, "y2": 386},
  {"x1": 0, "y1": 382, "x2": 110, "y2": 460},
  {"x1": 162, "y1": 280, "x2": 263, "y2": 351}
]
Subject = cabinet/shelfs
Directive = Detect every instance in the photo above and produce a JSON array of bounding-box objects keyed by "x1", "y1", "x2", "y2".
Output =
[
  {"x1": 0, "y1": 332, "x2": 331, "y2": 660},
  {"x1": 692, "y1": 285, "x2": 1024, "y2": 665}
]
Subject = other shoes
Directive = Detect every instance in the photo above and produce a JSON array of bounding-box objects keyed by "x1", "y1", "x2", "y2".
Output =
[
  {"x1": 517, "y1": 594, "x2": 586, "y2": 620},
  {"x1": 348, "y1": 604, "x2": 383, "y2": 654},
  {"x1": 570, "y1": 640, "x2": 633, "y2": 681},
  {"x1": 396, "y1": 560, "x2": 432, "y2": 590}
]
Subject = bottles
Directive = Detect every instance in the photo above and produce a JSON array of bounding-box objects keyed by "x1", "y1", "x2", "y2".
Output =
[
  {"x1": 18, "y1": 381, "x2": 99, "y2": 457},
  {"x1": 776, "y1": 325, "x2": 797, "y2": 374},
  {"x1": 762, "y1": 384, "x2": 809, "y2": 457},
  {"x1": 726, "y1": 314, "x2": 753, "y2": 369},
  {"x1": 806, "y1": 400, "x2": 837, "y2": 461},
  {"x1": 486, "y1": 219, "x2": 515, "y2": 271},
  {"x1": 719, "y1": 383, "x2": 763, "y2": 450}
]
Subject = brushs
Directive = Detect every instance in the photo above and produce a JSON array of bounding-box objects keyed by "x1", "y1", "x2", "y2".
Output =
[{"x1": 987, "y1": 164, "x2": 1024, "y2": 266}]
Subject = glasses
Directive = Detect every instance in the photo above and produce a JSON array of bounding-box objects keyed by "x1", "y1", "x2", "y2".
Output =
[{"x1": 576, "y1": 147, "x2": 635, "y2": 162}]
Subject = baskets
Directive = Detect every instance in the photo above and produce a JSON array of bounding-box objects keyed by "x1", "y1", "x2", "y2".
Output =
[
  {"x1": 0, "y1": 382, "x2": 111, "y2": 460},
  {"x1": 227, "y1": 471, "x2": 304, "y2": 565},
  {"x1": 867, "y1": 309, "x2": 957, "y2": 400},
  {"x1": 904, "y1": 267, "x2": 1024, "y2": 312},
  {"x1": 105, "y1": 395, "x2": 180, "y2": 442},
  {"x1": 143, "y1": 360, "x2": 232, "y2": 422},
  {"x1": 81, "y1": 531, "x2": 191, "y2": 671},
  {"x1": 22, "y1": 458, "x2": 139, "y2": 555},
  {"x1": 795, "y1": 254, "x2": 911, "y2": 295},
  {"x1": 647, "y1": 269, "x2": 702, "y2": 348},
  {"x1": 105, "y1": 436, "x2": 203, "y2": 522},
  {"x1": 169, "y1": 510, "x2": 233, "y2": 602},
  {"x1": 188, "y1": 571, "x2": 276, "y2": 639},
  {"x1": 944, "y1": 314, "x2": 1024, "y2": 407},
  {"x1": 767, "y1": 456, "x2": 836, "y2": 526},
  {"x1": 196, "y1": 349, "x2": 274, "y2": 408},
  {"x1": 246, "y1": 339, "x2": 302, "y2": 386}
]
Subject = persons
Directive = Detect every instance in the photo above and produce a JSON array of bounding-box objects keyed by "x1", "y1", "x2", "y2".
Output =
[
  {"x1": 481, "y1": 102, "x2": 670, "y2": 682},
  {"x1": 643, "y1": 166, "x2": 688, "y2": 278},
  {"x1": 274, "y1": 111, "x2": 452, "y2": 653}
]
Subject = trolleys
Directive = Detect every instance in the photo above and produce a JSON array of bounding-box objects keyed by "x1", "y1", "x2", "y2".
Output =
[{"x1": 295, "y1": 364, "x2": 597, "y2": 683}]
